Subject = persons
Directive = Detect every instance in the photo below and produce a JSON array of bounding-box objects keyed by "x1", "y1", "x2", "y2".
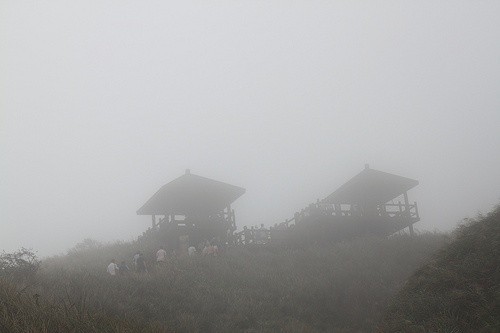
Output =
[
  {"x1": 106, "y1": 249, "x2": 147, "y2": 277},
  {"x1": 155, "y1": 246, "x2": 167, "y2": 264},
  {"x1": 188, "y1": 243, "x2": 218, "y2": 258}
]
